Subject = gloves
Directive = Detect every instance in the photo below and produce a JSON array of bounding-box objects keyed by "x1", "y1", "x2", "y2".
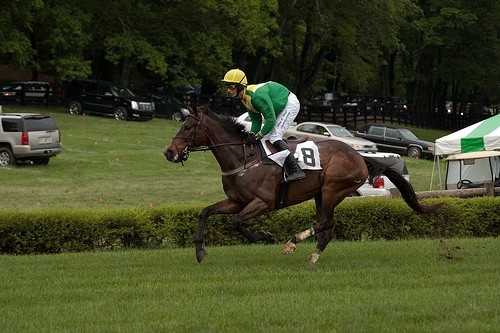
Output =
[{"x1": 254, "y1": 130, "x2": 263, "y2": 141}]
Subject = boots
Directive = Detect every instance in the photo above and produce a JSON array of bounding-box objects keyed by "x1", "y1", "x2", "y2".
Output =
[{"x1": 272, "y1": 140, "x2": 305, "y2": 181}]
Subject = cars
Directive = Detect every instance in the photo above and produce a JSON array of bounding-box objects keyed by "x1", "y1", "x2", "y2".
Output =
[
  {"x1": 233, "y1": 112, "x2": 298, "y2": 136},
  {"x1": 302, "y1": 90, "x2": 408, "y2": 120},
  {"x1": 167, "y1": 106, "x2": 191, "y2": 122},
  {"x1": 281, "y1": 121, "x2": 379, "y2": 154},
  {"x1": 435, "y1": 100, "x2": 497, "y2": 127}
]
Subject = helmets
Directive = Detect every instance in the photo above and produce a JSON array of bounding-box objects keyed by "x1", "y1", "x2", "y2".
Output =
[{"x1": 221, "y1": 68, "x2": 248, "y2": 87}]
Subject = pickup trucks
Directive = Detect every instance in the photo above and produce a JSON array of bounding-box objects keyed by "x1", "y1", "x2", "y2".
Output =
[{"x1": 349, "y1": 125, "x2": 443, "y2": 162}]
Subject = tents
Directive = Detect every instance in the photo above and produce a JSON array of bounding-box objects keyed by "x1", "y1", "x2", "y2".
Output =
[{"x1": 430, "y1": 113, "x2": 500, "y2": 193}]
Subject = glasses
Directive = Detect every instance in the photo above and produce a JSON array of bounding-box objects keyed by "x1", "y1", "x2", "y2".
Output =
[{"x1": 227, "y1": 85, "x2": 236, "y2": 90}]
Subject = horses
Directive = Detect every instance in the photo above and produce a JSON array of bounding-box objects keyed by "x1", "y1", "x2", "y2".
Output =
[{"x1": 162, "y1": 104, "x2": 449, "y2": 264}]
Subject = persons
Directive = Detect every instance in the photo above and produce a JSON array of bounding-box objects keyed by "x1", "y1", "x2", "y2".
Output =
[{"x1": 222, "y1": 69, "x2": 306, "y2": 183}]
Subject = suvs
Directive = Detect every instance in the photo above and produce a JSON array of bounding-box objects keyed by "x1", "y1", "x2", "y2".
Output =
[
  {"x1": 0, "y1": 113, "x2": 62, "y2": 167},
  {"x1": 61, "y1": 78, "x2": 156, "y2": 122},
  {"x1": 0, "y1": 80, "x2": 54, "y2": 105},
  {"x1": 345, "y1": 150, "x2": 409, "y2": 197}
]
